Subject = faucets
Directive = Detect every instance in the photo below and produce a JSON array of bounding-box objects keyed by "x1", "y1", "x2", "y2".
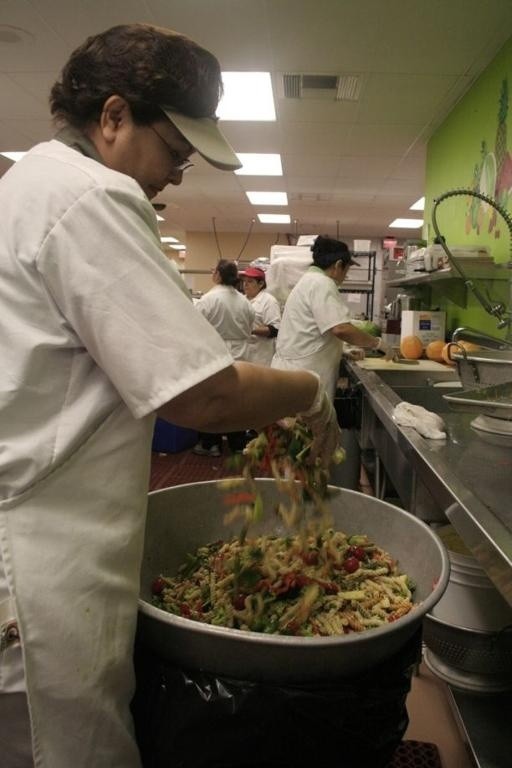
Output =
[
  {"x1": 432, "y1": 188, "x2": 512, "y2": 330},
  {"x1": 452, "y1": 327, "x2": 512, "y2": 341}
]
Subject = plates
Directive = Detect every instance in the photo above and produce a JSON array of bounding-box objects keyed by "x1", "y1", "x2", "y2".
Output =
[
  {"x1": 470, "y1": 426, "x2": 512, "y2": 450},
  {"x1": 423, "y1": 645, "x2": 511, "y2": 695},
  {"x1": 470, "y1": 413, "x2": 512, "y2": 435}
]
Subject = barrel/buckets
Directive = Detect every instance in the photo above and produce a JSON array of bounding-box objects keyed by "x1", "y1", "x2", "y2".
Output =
[{"x1": 431, "y1": 549, "x2": 512, "y2": 633}]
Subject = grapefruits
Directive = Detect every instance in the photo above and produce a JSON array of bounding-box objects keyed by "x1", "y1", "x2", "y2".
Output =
[
  {"x1": 426, "y1": 341, "x2": 480, "y2": 366},
  {"x1": 400, "y1": 336, "x2": 423, "y2": 359}
]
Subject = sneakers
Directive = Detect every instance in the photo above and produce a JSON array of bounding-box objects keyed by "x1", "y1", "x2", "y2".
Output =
[{"x1": 193, "y1": 441, "x2": 222, "y2": 457}]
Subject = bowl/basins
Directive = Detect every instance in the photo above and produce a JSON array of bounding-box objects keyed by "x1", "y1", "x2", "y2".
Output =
[{"x1": 138, "y1": 476, "x2": 451, "y2": 686}]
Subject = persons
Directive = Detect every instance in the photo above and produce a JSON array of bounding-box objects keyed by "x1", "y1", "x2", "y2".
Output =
[
  {"x1": 0, "y1": 23, "x2": 341, "y2": 767},
  {"x1": 238, "y1": 267, "x2": 281, "y2": 366},
  {"x1": 192, "y1": 259, "x2": 255, "y2": 457},
  {"x1": 270, "y1": 235, "x2": 398, "y2": 406}
]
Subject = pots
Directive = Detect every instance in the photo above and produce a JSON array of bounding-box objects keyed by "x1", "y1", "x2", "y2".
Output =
[{"x1": 448, "y1": 343, "x2": 512, "y2": 391}]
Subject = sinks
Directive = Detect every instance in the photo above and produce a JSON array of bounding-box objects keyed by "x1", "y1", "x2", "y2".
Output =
[
  {"x1": 389, "y1": 387, "x2": 464, "y2": 414},
  {"x1": 374, "y1": 369, "x2": 463, "y2": 387}
]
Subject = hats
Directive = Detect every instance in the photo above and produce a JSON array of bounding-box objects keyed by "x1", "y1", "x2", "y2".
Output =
[
  {"x1": 50, "y1": 21, "x2": 242, "y2": 170},
  {"x1": 314, "y1": 251, "x2": 361, "y2": 267},
  {"x1": 237, "y1": 267, "x2": 265, "y2": 280}
]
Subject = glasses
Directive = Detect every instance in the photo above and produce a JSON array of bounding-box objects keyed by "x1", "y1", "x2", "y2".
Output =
[{"x1": 144, "y1": 118, "x2": 195, "y2": 170}]
